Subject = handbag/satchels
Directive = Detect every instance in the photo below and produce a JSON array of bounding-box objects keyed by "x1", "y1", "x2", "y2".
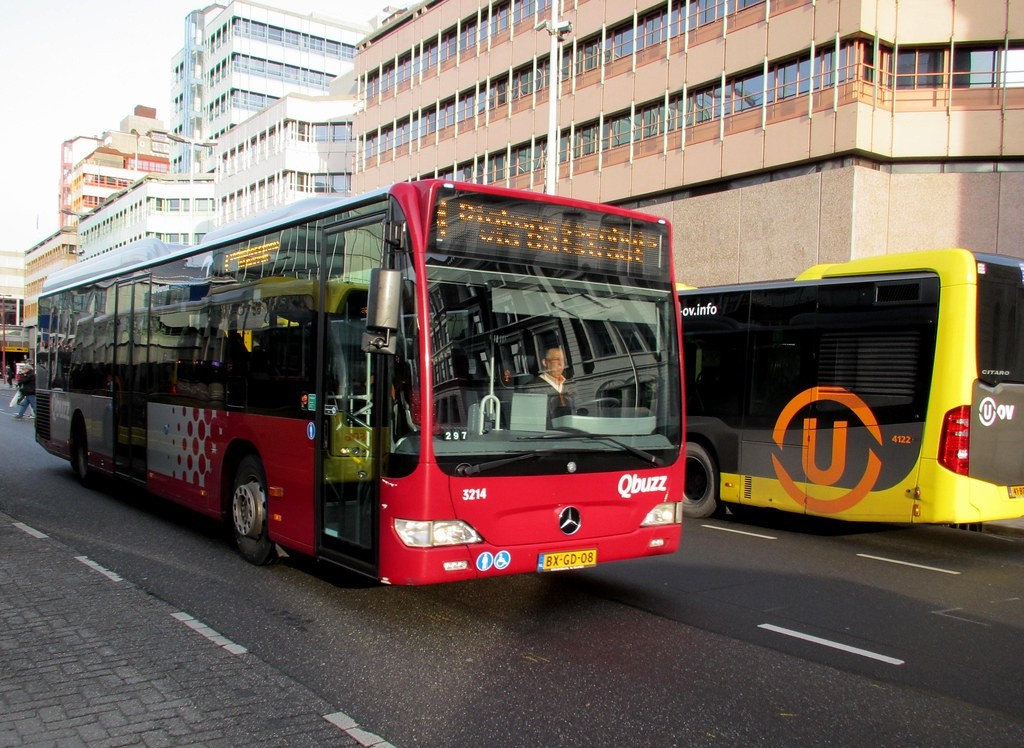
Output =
[{"x1": 10, "y1": 390, "x2": 25, "y2": 407}]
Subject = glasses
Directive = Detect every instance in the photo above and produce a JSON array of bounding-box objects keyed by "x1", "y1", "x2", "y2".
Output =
[{"x1": 545, "y1": 358, "x2": 565, "y2": 363}]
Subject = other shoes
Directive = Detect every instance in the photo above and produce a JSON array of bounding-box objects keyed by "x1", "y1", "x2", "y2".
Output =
[
  {"x1": 28, "y1": 416, "x2": 36, "y2": 419},
  {"x1": 13, "y1": 416, "x2": 23, "y2": 419}
]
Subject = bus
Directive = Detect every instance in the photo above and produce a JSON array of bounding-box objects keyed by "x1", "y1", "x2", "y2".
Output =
[
  {"x1": 68, "y1": 276, "x2": 393, "y2": 487},
  {"x1": 33, "y1": 180, "x2": 686, "y2": 586},
  {"x1": 672, "y1": 249, "x2": 1024, "y2": 524}
]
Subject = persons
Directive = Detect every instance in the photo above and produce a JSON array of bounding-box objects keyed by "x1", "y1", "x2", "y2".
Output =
[
  {"x1": 6, "y1": 365, "x2": 36, "y2": 417},
  {"x1": 524, "y1": 348, "x2": 570, "y2": 410}
]
